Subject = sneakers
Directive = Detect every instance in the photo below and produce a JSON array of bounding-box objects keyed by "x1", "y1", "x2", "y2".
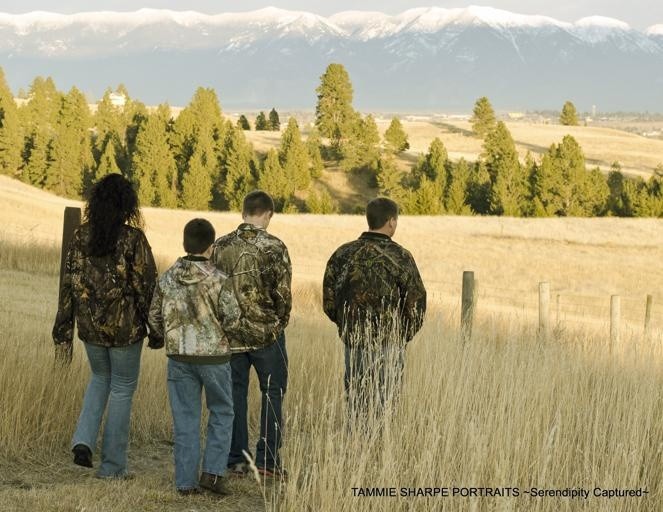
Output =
[
  {"x1": 230, "y1": 462, "x2": 290, "y2": 479},
  {"x1": 177, "y1": 472, "x2": 236, "y2": 496},
  {"x1": 73, "y1": 444, "x2": 94, "y2": 468}
]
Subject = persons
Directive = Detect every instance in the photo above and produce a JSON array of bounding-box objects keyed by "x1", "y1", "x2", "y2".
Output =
[
  {"x1": 210, "y1": 189, "x2": 292, "y2": 481},
  {"x1": 146, "y1": 217, "x2": 243, "y2": 497},
  {"x1": 51, "y1": 172, "x2": 160, "y2": 481},
  {"x1": 322, "y1": 197, "x2": 427, "y2": 420}
]
